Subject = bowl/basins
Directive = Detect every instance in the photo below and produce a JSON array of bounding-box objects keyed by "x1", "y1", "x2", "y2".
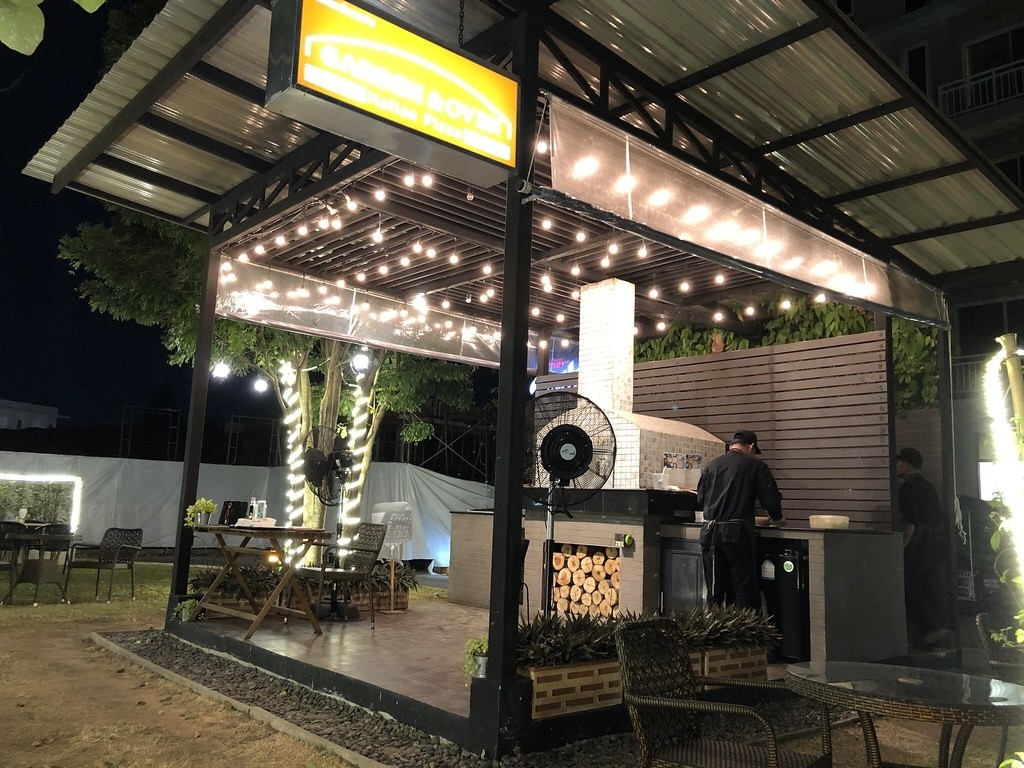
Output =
[
  {"x1": 754, "y1": 517, "x2": 771, "y2": 523},
  {"x1": 809, "y1": 515, "x2": 850, "y2": 529}
]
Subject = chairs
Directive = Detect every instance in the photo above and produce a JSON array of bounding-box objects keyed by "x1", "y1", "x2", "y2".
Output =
[
  {"x1": 976, "y1": 612, "x2": 1024, "y2": 768},
  {"x1": 284, "y1": 521, "x2": 388, "y2": 633},
  {"x1": 0, "y1": 518, "x2": 143, "y2": 607},
  {"x1": 615, "y1": 616, "x2": 832, "y2": 768}
]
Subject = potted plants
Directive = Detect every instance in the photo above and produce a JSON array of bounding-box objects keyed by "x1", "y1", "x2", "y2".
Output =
[
  {"x1": 191, "y1": 559, "x2": 421, "y2": 618},
  {"x1": 463, "y1": 630, "x2": 487, "y2": 686},
  {"x1": 516, "y1": 600, "x2": 786, "y2": 719}
]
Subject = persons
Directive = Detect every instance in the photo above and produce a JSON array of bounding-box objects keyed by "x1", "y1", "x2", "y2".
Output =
[
  {"x1": 665, "y1": 453, "x2": 701, "y2": 469},
  {"x1": 698, "y1": 430, "x2": 786, "y2": 619},
  {"x1": 895, "y1": 447, "x2": 947, "y2": 648}
]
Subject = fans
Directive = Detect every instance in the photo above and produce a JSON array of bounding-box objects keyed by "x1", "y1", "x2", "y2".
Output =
[
  {"x1": 303, "y1": 424, "x2": 367, "y2": 622},
  {"x1": 513, "y1": 391, "x2": 617, "y2": 619}
]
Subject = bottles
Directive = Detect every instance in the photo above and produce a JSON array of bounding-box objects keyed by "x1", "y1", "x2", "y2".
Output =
[
  {"x1": 257, "y1": 499, "x2": 267, "y2": 518},
  {"x1": 249, "y1": 497, "x2": 258, "y2": 520}
]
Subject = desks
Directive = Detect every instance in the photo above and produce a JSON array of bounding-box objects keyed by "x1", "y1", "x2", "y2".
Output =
[
  {"x1": 187, "y1": 524, "x2": 336, "y2": 641},
  {"x1": 5, "y1": 534, "x2": 83, "y2": 608},
  {"x1": 784, "y1": 661, "x2": 1024, "y2": 768}
]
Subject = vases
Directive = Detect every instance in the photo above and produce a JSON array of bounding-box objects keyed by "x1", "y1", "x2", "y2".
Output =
[
  {"x1": 197, "y1": 512, "x2": 212, "y2": 525},
  {"x1": 182, "y1": 606, "x2": 196, "y2": 622}
]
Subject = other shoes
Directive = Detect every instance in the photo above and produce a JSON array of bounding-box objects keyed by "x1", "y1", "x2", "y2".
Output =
[{"x1": 916, "y1": 628, "x2": 951, "y2": 650}]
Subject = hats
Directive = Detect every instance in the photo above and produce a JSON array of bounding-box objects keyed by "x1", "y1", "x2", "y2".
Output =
[
  {"x1": 896, "y1": 448, "x2": 924, "y2": 465},
  {"x1": 732, "y1": 430, "x2": 761, "y2": 455}
]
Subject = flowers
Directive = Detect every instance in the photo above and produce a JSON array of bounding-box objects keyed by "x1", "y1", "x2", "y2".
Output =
[
  {"x1": 171, "y1": 599, "x2": 199, "y2": 618},
  {"x1": 184, "y1": 497, "x2": 218, "y2": 528}
]
